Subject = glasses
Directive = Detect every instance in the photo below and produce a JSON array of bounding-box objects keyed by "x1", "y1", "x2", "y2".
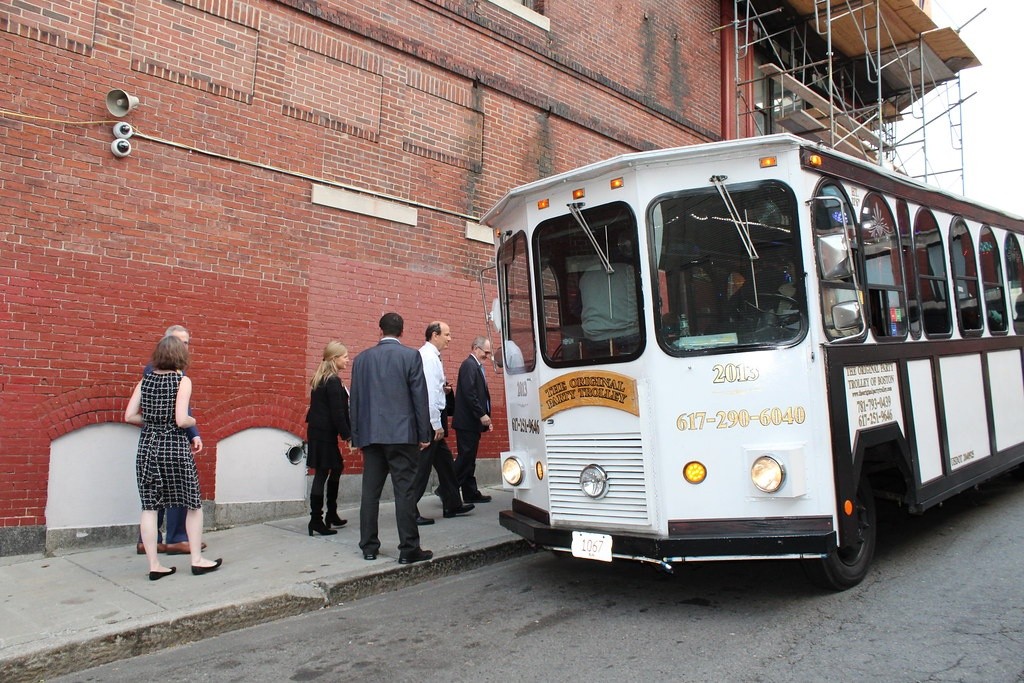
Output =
[{"x1": 477, "y1": 346, "x2": 489, "y2": 355}]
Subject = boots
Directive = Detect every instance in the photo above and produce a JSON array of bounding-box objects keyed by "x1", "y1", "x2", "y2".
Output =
[
  {"x1": 325, "y1": 492, "x2": 348, "y2": 528},
  {"x1": 308, "y1": 494, "x2": 337, "y2": 535}
]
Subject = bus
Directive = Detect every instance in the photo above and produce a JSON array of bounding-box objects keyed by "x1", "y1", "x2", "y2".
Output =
[{"x1": 478, "y1": 131, "x2": 1024, "y2": 593}]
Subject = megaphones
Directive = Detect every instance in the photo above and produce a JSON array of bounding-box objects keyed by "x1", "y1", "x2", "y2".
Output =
[{"x1": 106, "y1": 89, "x2": 140, "y2": 117}]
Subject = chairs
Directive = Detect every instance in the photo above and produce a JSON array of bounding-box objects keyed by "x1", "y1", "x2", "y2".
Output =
[{"x1": 906, "y1": 288, "x2": 1024, "y2": 337}]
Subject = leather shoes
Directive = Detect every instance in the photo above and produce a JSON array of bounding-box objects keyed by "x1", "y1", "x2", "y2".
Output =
[
  {"x1": 398, "y1": 548, "x2": 433, "y2": 565},
  {"x1": 166, "y1": 540, "x2": 206, "y2": 555},
  {"x1": 443, "y1": 504, "x2": 475, "y2": 518},
  {"x1": 416, "y1": 515, "x2": 434, "y2": 525},
  {"x1": 434, "y1": 486, "x2": 446, "y2": 504},
  {"x1": 137, "y1": 542, "x2": 167, "y2": 554},
  {"x1": 464, "y1": 494, "x2": 491, "y2": 502},
  {"x1": 364, "y1": 549, "x2": 379, "y2": 560}
]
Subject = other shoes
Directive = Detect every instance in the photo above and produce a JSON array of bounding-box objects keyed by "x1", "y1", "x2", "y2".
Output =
[
  {"x1": 149, "y1": 567, "x2": 176, "y2": 581},
  {"x1": 191, "y1": 558, "x2": 222, "y2": 575}
]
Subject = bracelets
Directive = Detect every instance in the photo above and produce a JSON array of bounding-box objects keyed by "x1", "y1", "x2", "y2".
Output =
[{"x1": 347, "y1": 438, "x2": 352, "y2": 442}]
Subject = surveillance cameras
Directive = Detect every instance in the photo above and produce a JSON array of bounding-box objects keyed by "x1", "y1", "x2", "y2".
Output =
[
  {"x1": 110, "y1": 138, "x2": 131, "y2": 157},
  {"x1": 113, "y1": 122, "x2": 133, "y2": 139}
]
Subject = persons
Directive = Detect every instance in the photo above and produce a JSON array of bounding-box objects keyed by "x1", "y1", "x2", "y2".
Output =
[
  {"x1": 578, "y1": 244, "x2": 639, "y2": 354},
  {"x1": 434, "y1": 335, "x2": 494, "y2": 503},
  {"x1": 305, "y1": 340, "x2": 357, "y2": 537},
  {"x1": 728, "y1": 258, "x2": 803, "y2": 334},
  {"x1": 416, "y1": 321, "x2": 475, "y2": 525},
  {"x1": 350, "y1": 313, "x2": 434, "y2": 565},
  {"x1": 136, "y1": 326, "x2": 207, "y2": 555},
  {"x1": 124, "y1": 335, "x2": 222, "y2": 581},
  {"x1": 986, "y1": 293, "x2": 1004, "y2": 327}
]
